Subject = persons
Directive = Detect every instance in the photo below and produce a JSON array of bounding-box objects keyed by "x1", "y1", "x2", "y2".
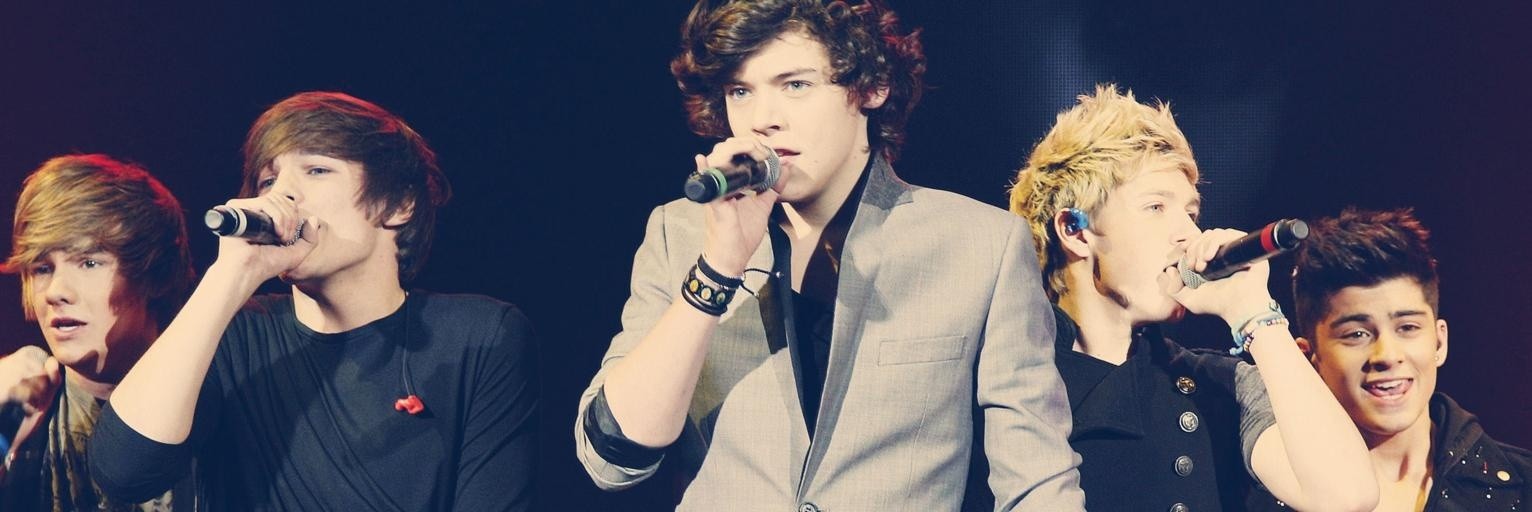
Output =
[
  {"x1": 1245, "y1": 203, "x2": 1532, "y2": 512},
  {"x1": 963, "y1": 82, "x2": 1380, "y2": 512},
  {"x1": 573, "y1": 0, "x2": 1087, "y2": 512},
  {"x1": 87, "y1": 93, "x2": 538, "y2": 512},
  {"x1": 0, "y1": 155, "x2": 216, "y2": 512}
]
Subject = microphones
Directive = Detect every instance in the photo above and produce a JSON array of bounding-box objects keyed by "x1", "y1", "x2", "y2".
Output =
[
  {"x1": 1175, "y1": 214, "x2": 1309, "y2": 293},
  {"x1": 201, "y1": 197, "x2": 309, "y2": 250},
  {"x1": 681, "y1": 141, "x2": 780, "y2": 204}
]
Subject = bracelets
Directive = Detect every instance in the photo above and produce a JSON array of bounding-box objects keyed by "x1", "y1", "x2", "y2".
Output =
[
  {"x1": 1229, "y1": 299, "x2": 1290, "y2": 356},
  {"x1": 681, "y1": 254, "x2": 783, "y2": 315}
]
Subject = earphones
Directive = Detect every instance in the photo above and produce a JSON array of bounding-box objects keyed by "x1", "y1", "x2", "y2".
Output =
[{"x1": 1061, "y1": 204, "x2": 1087, "y2": 236}]
